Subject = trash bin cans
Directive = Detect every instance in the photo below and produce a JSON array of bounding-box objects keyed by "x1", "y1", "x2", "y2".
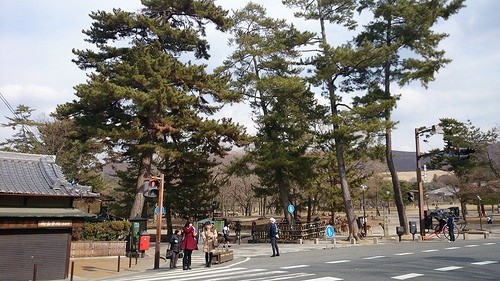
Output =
[{"x1": 129, "y1": 213, "x2": 148, "y2": 235}]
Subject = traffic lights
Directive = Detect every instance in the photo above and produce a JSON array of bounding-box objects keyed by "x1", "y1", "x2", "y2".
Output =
[
  {"x1": 149, "y1": 178, "x2": 160, "y2": 198},
  {"x1": 407, "y1": 192, "x2": 414, "y2": 202}
]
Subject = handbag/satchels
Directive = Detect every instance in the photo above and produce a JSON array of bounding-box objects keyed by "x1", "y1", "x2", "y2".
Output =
[
  {"x1": 179, "y1": 253, "x2": 183, "y2": 258},
  {"x1": 214, "y1": 239, "x2": 218, "y2": 247}
]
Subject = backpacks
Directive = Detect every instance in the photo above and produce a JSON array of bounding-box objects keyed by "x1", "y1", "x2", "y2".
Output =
[
  {"x1": 166, "y1": 249, "x2": 173, "y2": 259},
  {"x1": 237, "y1": 223, "x2": 241, "y2": 231}
]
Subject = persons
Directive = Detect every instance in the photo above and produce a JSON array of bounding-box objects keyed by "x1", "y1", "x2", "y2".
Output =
[
  {"x1": 269, "y1": 217, "x2": 280, "y2": 257},
  {"x1": 169, "y1": 229, "x2": 183, "y2": 269},
  {"x1": 223, "y1": 220, "x2": 256, "y2": 243},
  {"x1": 180, "y1": 221, "x2": 197, "y2": 270},
  {"x1": 202, "y1": 222, "x2": 217, "y2": 267},
  {"x1": 447, "y1": 211, "x2": 455, "y2": 243}
]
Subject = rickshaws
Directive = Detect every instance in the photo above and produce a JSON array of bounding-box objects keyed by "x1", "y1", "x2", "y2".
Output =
[{"x1": 423, "y1": 209, "x2": 459, "y2": 242}]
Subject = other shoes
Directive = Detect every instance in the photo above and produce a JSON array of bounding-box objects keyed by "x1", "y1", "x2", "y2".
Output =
[
  {"x1": 184, "y1": 266, "x2": 192, "y2": 269},
  {"x1": 271, "y1": 255, "x2": 275, "y2": 257},
  {"x1": 206, "y1": 264, "x2": 211, "y2": 267},
  {"x1": 170, "y1": 266, "x2": 178, "y2": 269},
  {"x1": 276, "y1": 254, "x2": 279, "y2": 256}
]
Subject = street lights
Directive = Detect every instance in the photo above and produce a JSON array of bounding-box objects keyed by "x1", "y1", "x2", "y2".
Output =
[{"x1": 360, "y1": 184, "x2": 368, "y2": 236}]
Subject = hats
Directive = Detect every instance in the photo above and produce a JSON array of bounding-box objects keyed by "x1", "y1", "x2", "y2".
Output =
[
  {"x1": 270, "y1": 218, "x2": 275, "y2": 223},
  {"x1": 203, "y1": 221, "x2": 210, "y2": 225}
]
